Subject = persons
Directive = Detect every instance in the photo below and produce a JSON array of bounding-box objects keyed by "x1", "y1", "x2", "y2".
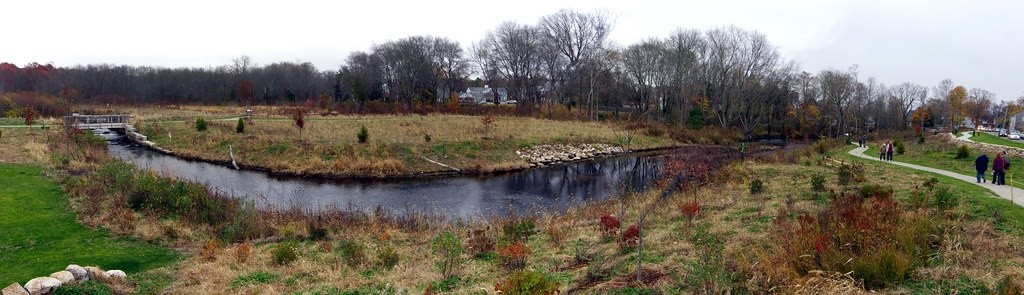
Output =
[
  {"x1": 975, "y1": 150, "x2": 989, "y2": 183},
  {"x1": 880, "y1": 143, "x2": 886, "y2": 161},
  {"x1": 1001, "y1": 151, "x2": 1010, "y2": 185},
  {"x1": 863, "y1": 135, "x2": 866, "y2": 148},
  {"x1": 887, "y1": 141, "x2": 894, "y2": 161},
  {"x1": 991, "y1": 152, "x2": 1004, "y2": 185},
  {"x1": 858, "y1": 136, "x2": 862, "y2": 147}
]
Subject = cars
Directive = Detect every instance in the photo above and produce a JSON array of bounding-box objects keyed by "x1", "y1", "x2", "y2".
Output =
[
  {"x1": 999, "y1": 131, "x2": 1008, "y2": 137},
  {"x1": 846, "y1": 132, "x2": 853, "y2": 139},
  {"x1": 507, "y1": 99, "x2": 518, "y2": 104},
  {"x1": 1010, "y1": 132, "x2": 1020, "y2": 140}
]
